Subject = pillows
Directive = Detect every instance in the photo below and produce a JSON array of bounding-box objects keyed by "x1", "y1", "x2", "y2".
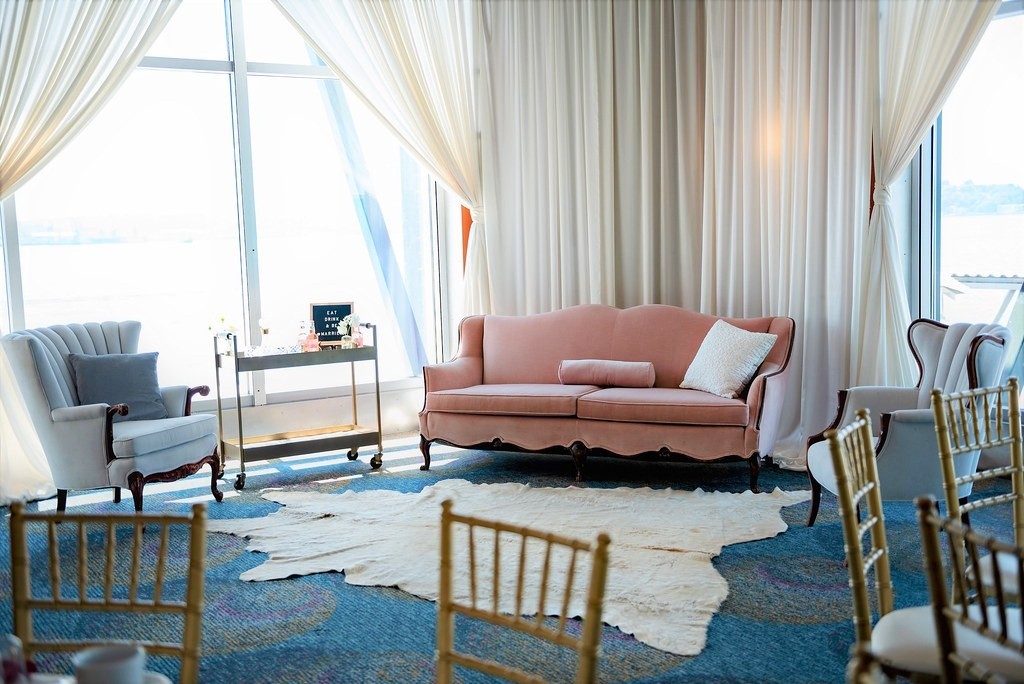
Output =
[
  {"x1": 679, "y1": 319, "x2": 778, "y2": 400},
  {"x1": 69, "y1": 352, "x2": 168, "y2": 424},
  {"x1": 558, "y1": 359, "x2": 655, "y2": 388}
]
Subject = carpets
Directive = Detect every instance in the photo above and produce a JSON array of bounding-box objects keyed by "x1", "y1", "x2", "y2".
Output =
[{"x1": 207, "y1": 478, "x2": 822, "y2": 655}]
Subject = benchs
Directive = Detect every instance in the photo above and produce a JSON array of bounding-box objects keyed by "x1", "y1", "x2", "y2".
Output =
[{"x1": 418, "y1": 303, "x2": 795, "y2": 494}]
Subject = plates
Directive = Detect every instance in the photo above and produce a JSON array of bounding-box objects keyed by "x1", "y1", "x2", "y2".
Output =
[
  {"x1": 67, "y1": 671, "x2": 172, "y2": 684},
  {"x1": 30, "y1": 673, "x2": 67, "y2": 684}
]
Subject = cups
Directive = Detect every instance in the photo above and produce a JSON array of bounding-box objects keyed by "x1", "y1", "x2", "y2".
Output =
[{"x1": 72, "y1": 645, "x2": 144, "y2": 684}]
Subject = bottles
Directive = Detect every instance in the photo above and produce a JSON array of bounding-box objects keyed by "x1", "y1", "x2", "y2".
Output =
[
  {"x1": 298, "y1": 321, "x2": 307, "y2": 352},
  {"x1": 0, "y1": 635, "x2": 26, "y2": 684},
  {"x1": 307, "y1": 320, "x2": 319, "y2": 352},
  {"x1": 258, "y1": 318, "x2": 278, "y2": 356}
]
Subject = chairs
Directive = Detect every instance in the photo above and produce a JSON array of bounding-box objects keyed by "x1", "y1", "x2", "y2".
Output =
[
  {"x1": 436, "y1": 499, "x2": 611, "y2": 684},
  {"x1": 807, "y1": 318, "x2": 1024, "y2": 684},
  {"x1": 0, "y1": 320, "x2": 224, "y2": 532},
  {"x1": 9, "y1": 499, "x2": 208, "y2": 684}
]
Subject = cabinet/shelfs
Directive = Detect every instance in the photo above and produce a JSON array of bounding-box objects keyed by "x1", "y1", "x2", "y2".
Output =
[{"x1": 214, "y1": 323, "x2": 383, "y2": 489}]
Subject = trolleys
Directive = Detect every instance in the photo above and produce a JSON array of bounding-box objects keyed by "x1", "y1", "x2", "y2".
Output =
[{"x1": 213, "y1": 322, "x2": 383, "y2": 490}]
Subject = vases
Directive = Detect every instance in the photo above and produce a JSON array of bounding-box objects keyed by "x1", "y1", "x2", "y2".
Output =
[{"x1": 342, "y1": 333, "x2": 362, "y2": 349}]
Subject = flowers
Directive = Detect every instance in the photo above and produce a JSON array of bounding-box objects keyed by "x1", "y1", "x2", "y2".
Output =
[{"x1": 337, "y1": 313, "x2": 360, "y2": 335}]
